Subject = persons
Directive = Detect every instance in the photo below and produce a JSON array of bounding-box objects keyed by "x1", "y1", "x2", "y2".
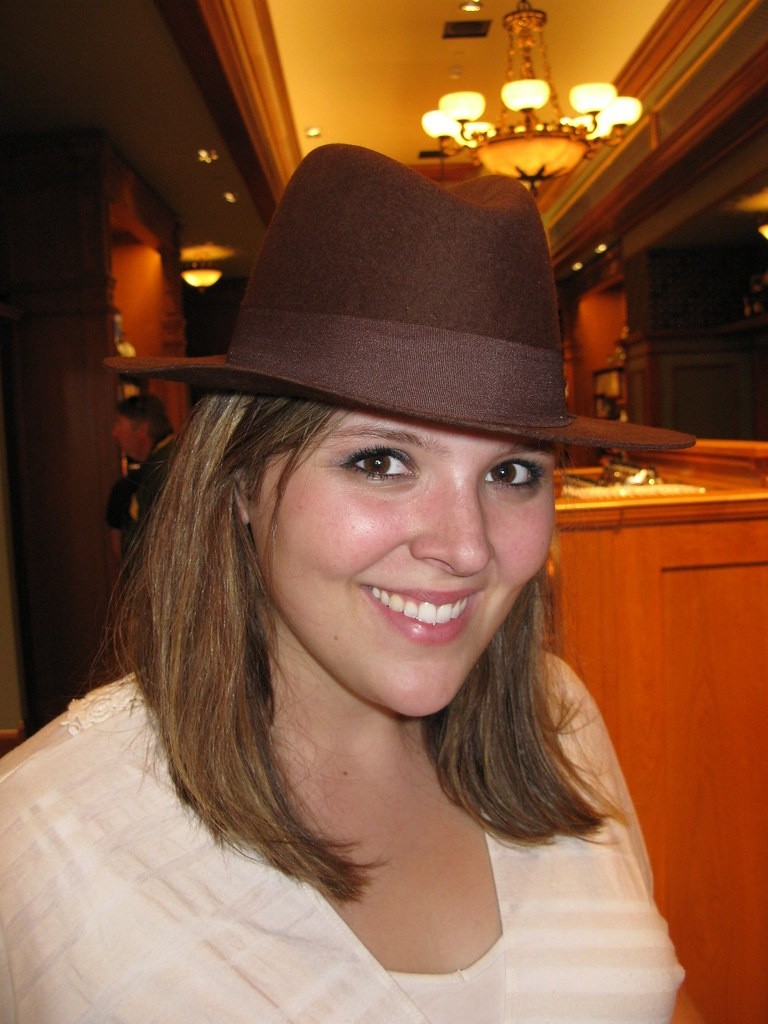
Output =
[
  {"x1": 0, "y1": 143, "x2": 686, "y2": 1024},
  {"x1": 112, "y1": 393, "x2": 185, "y2": 507},
  {"x1": 107, "y1": 455, "x2": 145, "y2": 568}
]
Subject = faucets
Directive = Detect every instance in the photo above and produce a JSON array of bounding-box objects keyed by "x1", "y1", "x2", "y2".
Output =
[{"x1": 625, "y1": 468, "x2": 658, "y2": 485}]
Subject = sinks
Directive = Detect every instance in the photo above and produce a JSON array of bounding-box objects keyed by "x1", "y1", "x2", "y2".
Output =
[{"x1": 569, "y1": 485, "x2": 707, "y2": 498}]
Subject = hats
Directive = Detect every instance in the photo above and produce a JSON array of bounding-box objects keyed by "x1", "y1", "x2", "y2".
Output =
[{"x1": 103, "y1": 141, "x2": 699, "y2": 450}]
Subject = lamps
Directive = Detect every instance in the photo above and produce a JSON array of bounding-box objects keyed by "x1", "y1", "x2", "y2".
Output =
[
  {"x1": 420, "y1": 0, "x2": 643, "y2": 193},
  {"x1": 177, "y1": 260, "x2": 226, "y2": 294}
]
